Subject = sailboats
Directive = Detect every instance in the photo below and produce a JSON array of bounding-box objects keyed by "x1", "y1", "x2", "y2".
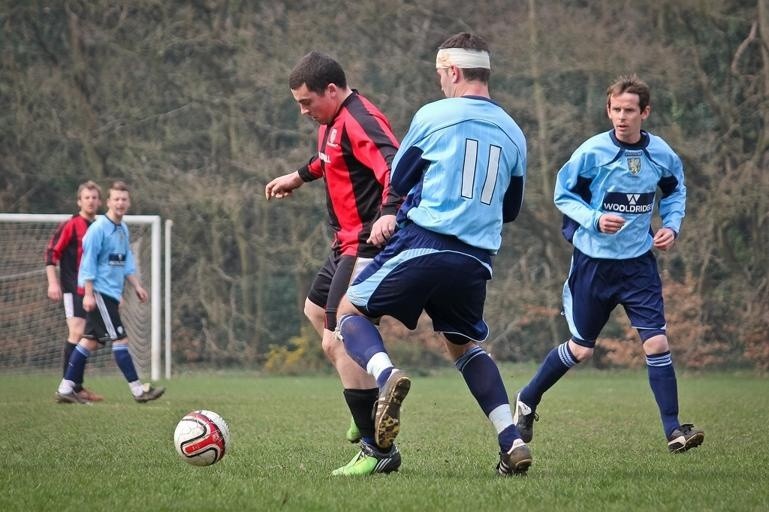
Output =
[{"x1": 174, "y1": 410, "x2": 230, "y2": 466}]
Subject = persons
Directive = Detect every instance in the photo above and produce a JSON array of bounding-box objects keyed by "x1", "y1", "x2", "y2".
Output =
[
  {"x1": 44, "y1": 179, "x2": 124, "y2": 400},
  {"x1": 514, "y1": 75, "x2": 705, "y2": 452},
  {"x1": 53, "y1": 180, "x2": 165, "y2": 405},
  {"x1": 336, "y1": 33, "x2": 532, "y2": 476},
  {"x1": 265, "y1": 51, "x2": 411, "y2": 477}
]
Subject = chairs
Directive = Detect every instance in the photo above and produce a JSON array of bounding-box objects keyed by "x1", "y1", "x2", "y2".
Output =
[
  {"x1": 132, "y1": 384, "x2": 165, "y2": 403},
  {"x1": 667, "y1": 424, "x2": 704, "y2": 454},
  {"x1": 496, "y1": 438, "x2": 532, "y2": 474},
  {"x1": 330, "y1": 440, "x2": 401, "y2": 475},
  {"x1": 347, "y1": 418, "x2": 361, "y2": 443},
  {"x1": 372, "y1": 371, "x2": 411, "y2": 448},
  {"x1": 79, "y1": 389, "x2": 103, "y2": 400},
  {"x1": 56, "y1": 392, "x2": 88, "y2": 405},
  {"x1": 513, "y1": 391, "x2": 540, "y2": 443}
]
